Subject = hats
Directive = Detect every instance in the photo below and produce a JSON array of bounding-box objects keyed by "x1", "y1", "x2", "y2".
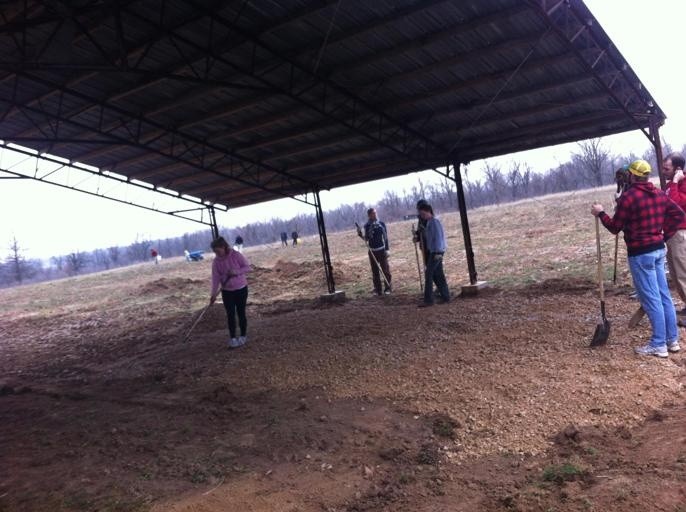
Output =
[{"x1": 627, "y1": 159, "x2": 651, "y2": 177}]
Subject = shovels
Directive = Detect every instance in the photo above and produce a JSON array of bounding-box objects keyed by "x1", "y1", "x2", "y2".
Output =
[{"x1": 590, "y1": 200, "x2": 611, "y2": 347}]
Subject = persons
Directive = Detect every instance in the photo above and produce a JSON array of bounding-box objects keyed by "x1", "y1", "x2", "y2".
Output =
[
  {"x1": 151, "y1": 247, "x2": 159, "y2": 264},
  {"x1": 280, "y1": 230, "x2": 288, "y2": 247},
  {"x1": 184, "y1": 248, "x2": 191, "y2": 261},
  {"x1": 419, "y1": 205, "x2": 450, "y2": 307},
  {"x1": 663, "y1": 152, "x2": 686, "y2": 328},
  {"x1": 357, "y1": 209, "x2": 392, "y2": 295},
  {"x1": 291, "y1": 230, "x2": 298, "y2": 246},
  {"x1": 614, "y1": 166, "x2": 629, "y2": 196},
  {"x1": 591, "y1": 160, "x2": 681, "y2": 359},
  {"x1": 209, "y1": 237, "x2": 250, "y2": 348},
  {"x1": 234, "y1": 235, "x2": 244, "y2": 253},
  {"x1": 412, "y1": 201, "x2": 443, "y2": 300}
]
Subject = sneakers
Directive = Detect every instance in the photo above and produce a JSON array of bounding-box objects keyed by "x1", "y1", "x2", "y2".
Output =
[
  {"x1": 667, "y1": 341, "x2": 680, "y2": 352},
  {"x1": 238, "y1": 336, "x2": 246, "y2": 345},
  {"x1": 634, "y1": 344, "x2": 668, "y2": 357},
  {"x1": 230, "y1": 339, "x2": 238, "y2": 348}
]
